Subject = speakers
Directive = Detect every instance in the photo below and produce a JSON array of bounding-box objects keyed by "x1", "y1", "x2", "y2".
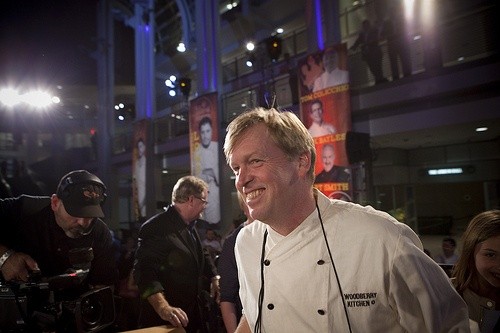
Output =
[{"x1": 345, "y1": 132, "x2": 370, "y2": 163}]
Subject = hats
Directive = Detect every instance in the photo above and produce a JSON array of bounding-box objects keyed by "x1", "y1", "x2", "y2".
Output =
[{"x1": 57, "y1": 169, "x2": 104, "y2": 217}]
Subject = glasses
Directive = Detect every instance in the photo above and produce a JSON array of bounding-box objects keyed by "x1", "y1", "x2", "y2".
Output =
[{"x1": 194, "y1": 195, "x2": 208, "y2": 205}]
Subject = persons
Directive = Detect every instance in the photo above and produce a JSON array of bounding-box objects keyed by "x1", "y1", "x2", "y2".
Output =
[
  {"x1": 201, "y1": 228, "x2": 224, "y2": 254},
  {"x1": 195, "y1": 117, "x2": 219, "y2": 225},
  {"x1": 283, "y1": 52, "x2": 300, "y2": 103},
  {"x1": 128, "y1": 176, "x2": 221, "y2": 333},
  {"x1": 313, "y1": 144, "x2": 351, "y2": 183},
  {"x1": 134, "y1": 137, "x2": 148, "y2": 218},
  {"x1": 222, "y1": 94, "x2": 471, "y2": 333},
  {"x1": 308, "y1": 99, "x2": 336, "y2": 138},
  {"x1": 450, "y1": 208, "x2": 500, "y2": 333},
  {"x1": 349, "y1": 6, "x2": 414, "y2": 87},
  {"x1": 112, "y1": 221, "x2": 144, "y2": 330},
  {"x1": 218, "y1": 188, "x2": 254, "y2": 333},
  {"x1": 434, "y1": 238, "x2": 459, "y2": 265},
  {"x1": 313, "y1": 47, "x2": 349, "y2": 92},
  {"x1": 0, "y1": 169, "x2": 114, "y2": 333}
]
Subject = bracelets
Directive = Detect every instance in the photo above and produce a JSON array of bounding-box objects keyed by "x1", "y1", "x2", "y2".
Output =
[
  {"x1": 0, "y1": 248, "x2": 14, "y2": 269},
  {"x1": 210, "y1": 274, "x2": 221, "y2": 280}
]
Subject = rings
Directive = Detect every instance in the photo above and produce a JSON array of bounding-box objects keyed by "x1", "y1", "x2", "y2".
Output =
[{"x1": 169, "y1": 315, "x2": 176, "y2": 320}]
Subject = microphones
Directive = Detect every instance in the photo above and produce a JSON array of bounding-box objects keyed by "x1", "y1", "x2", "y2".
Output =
[{"x1": 78, "y1": 225, "x2": 95, "y2": 236}]
